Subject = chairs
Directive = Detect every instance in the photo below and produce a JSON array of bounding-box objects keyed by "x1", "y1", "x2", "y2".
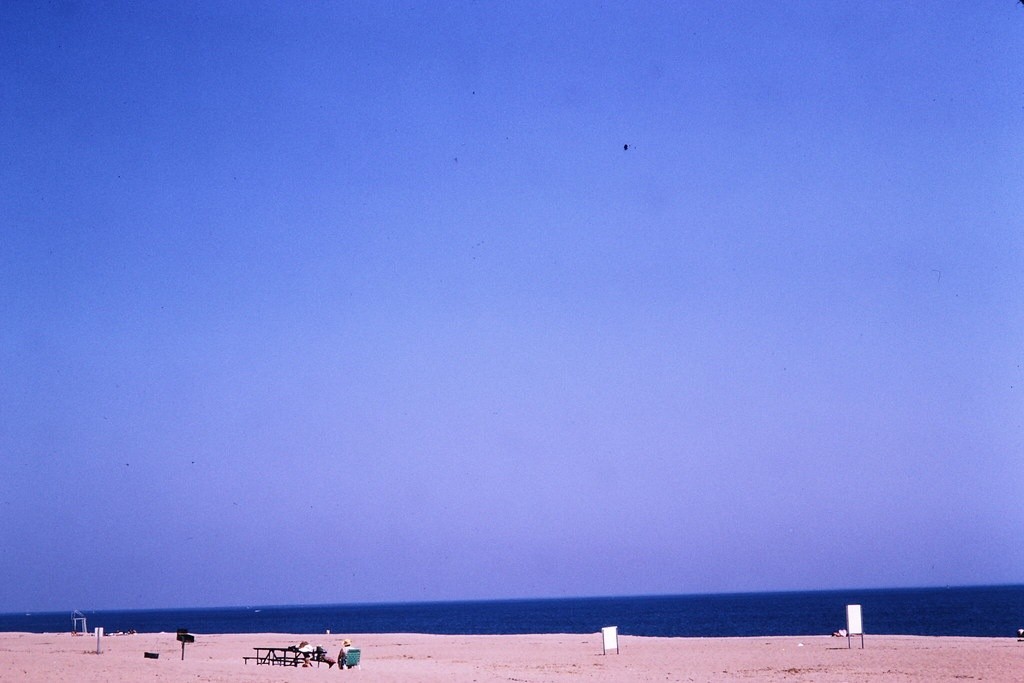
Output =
[{"x1": 341, "y1": 649, "x2": 361, "y2": 669}]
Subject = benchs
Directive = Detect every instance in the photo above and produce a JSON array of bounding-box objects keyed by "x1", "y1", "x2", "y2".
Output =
[
  {"x1": 276, "y1": 657, "x2": 326, "y2": 664},
  {"x1": 243, "y1": 656, "x2": 266, "y2": 665},
  {"x1": 268, "y1": 658, "x2": 304, "y2": 666}
]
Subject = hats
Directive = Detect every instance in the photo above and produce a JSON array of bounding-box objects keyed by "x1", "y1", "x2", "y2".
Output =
[{"x1": 343, "y1": 639, "x2": 351, "y2": 645}]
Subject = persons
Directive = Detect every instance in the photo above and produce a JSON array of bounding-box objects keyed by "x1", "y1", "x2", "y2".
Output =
[
  {"x1": 832, "y1": 632, "x2": 856, "y2": 638},
  {"x1": 298, "y1": 641, "x2": 314, "y2": 668},
  {"x1": 337, "y1": 639, "x2": 355, "y2": 670}
]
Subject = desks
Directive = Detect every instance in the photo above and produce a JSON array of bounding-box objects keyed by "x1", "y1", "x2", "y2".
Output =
[{"x1": 254, "y1": 648, "x2": 326, "y2": 668}]
